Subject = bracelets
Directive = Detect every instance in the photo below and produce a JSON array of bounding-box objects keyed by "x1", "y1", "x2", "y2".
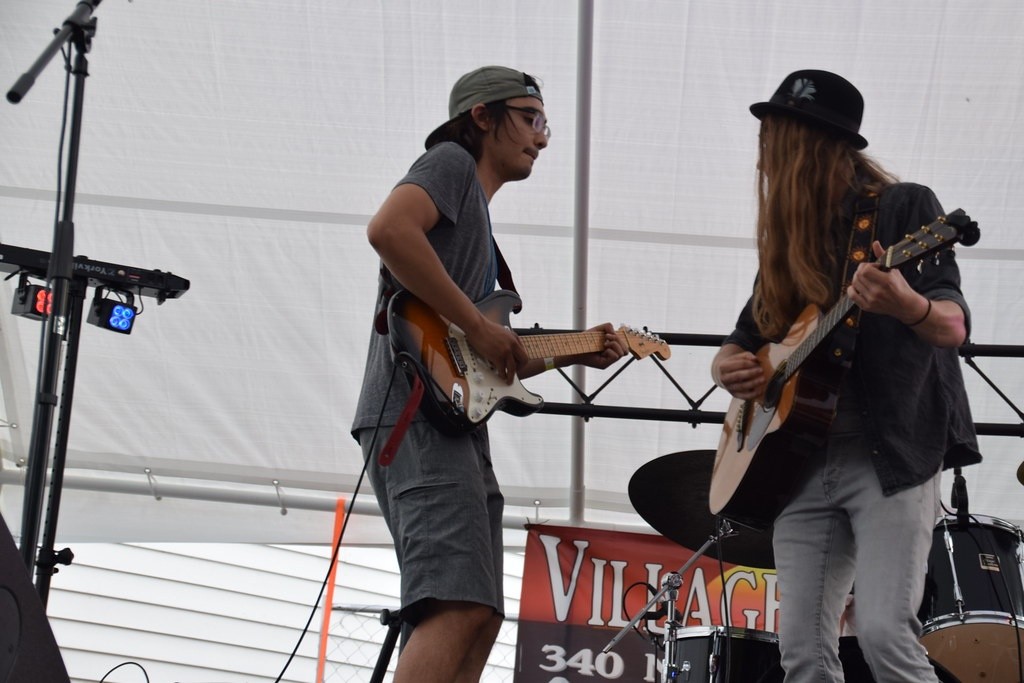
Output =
[
  {"x1": 907, "y1": 299, "x2": 931, "y2": 327},
  {"x1": 543, "y1": 357, "x2": 555, "y2": 371}
]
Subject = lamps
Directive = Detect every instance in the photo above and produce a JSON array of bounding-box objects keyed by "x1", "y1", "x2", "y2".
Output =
[
  {"x1": 86, "y1": 285, "x2": 137, "y2": 335},
  {"x1": 10, "y1": 270, "x2": 55, "y2": 320}
]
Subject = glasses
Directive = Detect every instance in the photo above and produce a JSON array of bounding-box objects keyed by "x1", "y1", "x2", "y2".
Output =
[{"x1": 507, "y1": 104, "x2": 551, "y2": 140}]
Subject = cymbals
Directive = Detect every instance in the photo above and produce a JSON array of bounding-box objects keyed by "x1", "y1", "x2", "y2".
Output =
[{"x1": 627, "y1": 448, "x2": 779, "y2": 571}]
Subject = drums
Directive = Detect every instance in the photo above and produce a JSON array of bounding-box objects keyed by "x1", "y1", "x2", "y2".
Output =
[
  {"x1": 913, "y1": 514, "x2": 1024, "y2": 682},
  {"x1": 671, "y1": 624, "x2": 786, "y2": 683}
]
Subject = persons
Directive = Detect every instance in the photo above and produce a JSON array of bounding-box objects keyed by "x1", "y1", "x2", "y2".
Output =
[
  {"x1": 349, "y1": 64, "x2": 625, "y2": 682},
  {"x1": 709, "y1": 67, "x2": 970, "y2": 683}
]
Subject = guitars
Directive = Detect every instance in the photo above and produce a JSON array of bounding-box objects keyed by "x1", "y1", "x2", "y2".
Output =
[
  {"x1": 708, "y1": 206, "x2": 982, "y2": 534},
  {"x1": 384, "y1": 288, "x2": 671, "y2": 436}
]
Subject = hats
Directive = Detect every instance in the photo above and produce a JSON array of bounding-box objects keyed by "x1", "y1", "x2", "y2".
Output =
[
  {"x1": 425, "y1": 67, "x2": 542, "y2": 151},
  {"x1": 749, "y1": 69, "x2": 868, "y2": 153}
]
